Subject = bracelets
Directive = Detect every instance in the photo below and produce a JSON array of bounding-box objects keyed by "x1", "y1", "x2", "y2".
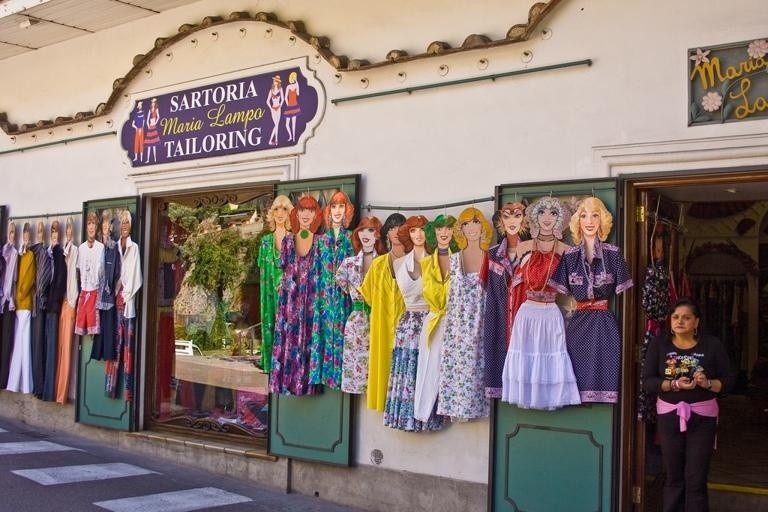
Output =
[{"x1": 670, "y1": 379, "x2": 680, "y2": 391}]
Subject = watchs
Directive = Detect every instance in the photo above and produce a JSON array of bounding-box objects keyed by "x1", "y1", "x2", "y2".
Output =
[{"x1": 707, "y1": 380, "x2": 713, "y2": 390}]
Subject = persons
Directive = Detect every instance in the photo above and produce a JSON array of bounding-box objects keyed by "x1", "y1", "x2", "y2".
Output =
[
  {"x1": 266, "y1": 76, "x2": 285, "y2": 145},
  {"x1": 640, "y1": 234, "x2": 690, "y2": 420},
  {"x1": 284, "y1": 73, "x2": 301, "y2": 143},
  {"x1": 131, "y1": 98, "x2": 145, "y2": 164},
  {"x1": 0, "y1": 210, "x2": 142, "y2": 406},
  {"x1": 642, "y1": 302, "x2": 727, "y2": 512},
  {"x1": 144, "y1": 98, "x2": 160, "y2": 163}
]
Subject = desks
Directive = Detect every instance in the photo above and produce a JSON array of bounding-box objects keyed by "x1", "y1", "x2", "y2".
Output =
[{"x1": 171, "y1": 351, "x2": 269, "y2": 396}]
